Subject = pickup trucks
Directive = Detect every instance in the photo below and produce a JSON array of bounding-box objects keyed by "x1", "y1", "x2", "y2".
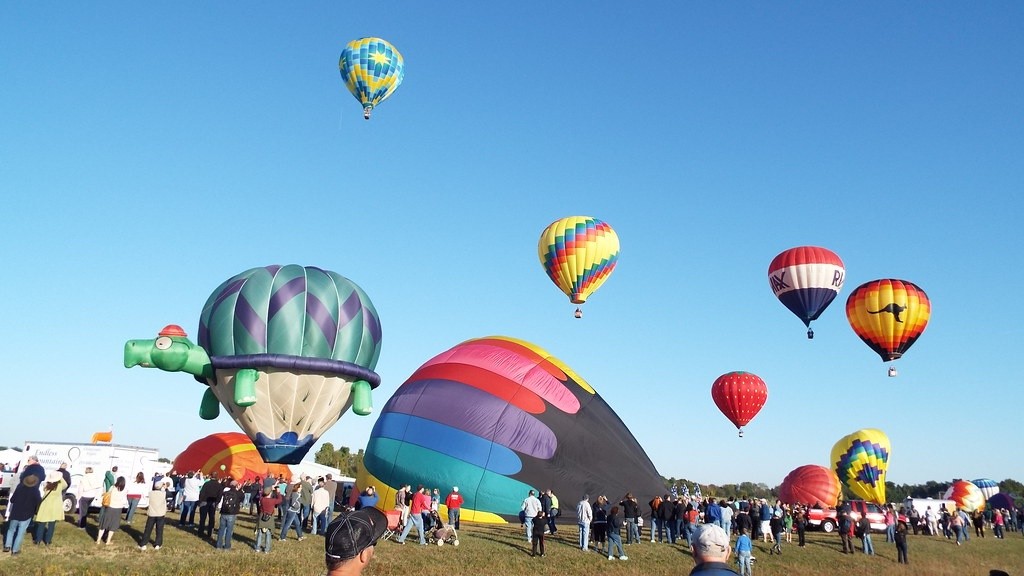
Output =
[{"x1": 808, "y1": 501, "x2": 910, "y2": 533}]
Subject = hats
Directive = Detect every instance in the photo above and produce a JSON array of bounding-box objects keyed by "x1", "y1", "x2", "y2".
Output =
[
  {"x1": 21, "y1": 474, "x2": 38, "y2": 489},
  {"x1": 324, "y1": 506, "x2": 390, "y2": 561},
  {"x1": 44, "y1": 469, "x2": 64, "y2": 484},
  {"x1": 691, "y1": 522, "x2": 730, "y2": 548},
  {"x1": 155, "y1": 482, "x2": 163, "y2": 489},
  {"x1": 453, "y1": 487, "x2": 459, "y2": 492}
]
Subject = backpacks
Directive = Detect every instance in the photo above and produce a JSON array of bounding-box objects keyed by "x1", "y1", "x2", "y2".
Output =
[{"x1": 101, "y1": 490, "x2": 112, "y2": 508}]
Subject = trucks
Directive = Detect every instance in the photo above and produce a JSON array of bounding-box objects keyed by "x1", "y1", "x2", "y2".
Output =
[{"x1": 0, "y1": 439, "x2": 160, "y2": 514}]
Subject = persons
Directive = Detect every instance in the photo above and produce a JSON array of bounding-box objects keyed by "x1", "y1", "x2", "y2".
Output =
[
  {"x1": 139, "y1": 481, "x2": 166, "y2": 551},
  {"x1": 254, "y1": 487, "x2": 283, "y2": 553},
  {"x1": 104, "y1": 466, "x2": 118, "y2": 493},
  {"x1": 689, "y1": 523, "x2": 743, "y2": 576},
  {"x1": 125, "y1": 472, "x2": 145, "y2": 524},
  {"x1": 325, "y1": 506, "x2": 388, "y2": 576},
  {"x1": 577, "y1": 493, "x2": 643, "y2": 552},
  {"x1": 649, "y1": 494, "x2": 824, "y2": 560},
  {"x1": 521, "y1": 489, "x2": 559, "y2": 543},
  {"x1": 151, "y1": 466, "x2": 337, "y2": 542},
  {"x1": 607, "y1": 507, "x2": 628, "y2": 560},
  {"x1": 95, "y1": 476, "x2": 128, "y2": 545},
  {"x1": 836, "y1": 501, "x2": 874, "y2": 555},
  {"x1": 394, "y1": 483, "x2": 464, "y2": 545},
  {"x1": 883, "y1": 503, "x2": 1024, "y2": 545},
  {"x1": 531, "y1": 511, "x2": 547, "y2": 558},
  {"x1": 358, "y1": 485, "x2": 379, "y2": 510},
  {"x1": 4, "y1": 455, "x2": 71, "y2": 556},
  {"x1": 733, "y1": 528, "x2": 751, "y2": 576},
  {"x1": 894, "y1": 523, "x2": 909, "y2": 564},
  {"x1": 216, "y1": 480, "x2": 245, "y2": 550},
  {"x1": 77, "y1": 466, "x2": 97, "y2": 529},
  {"x1": 341, "y1": 485, "x2": 353, "y2": 506}
]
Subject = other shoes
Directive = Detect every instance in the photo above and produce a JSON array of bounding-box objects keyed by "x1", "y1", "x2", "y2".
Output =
[
  {"x1": 770, "y1": 547, "x2": 774, "y2": 555},
  {"x1": 155, "y1": 545, "x2": 162, "y2": 550},
  {"x1": 620, "y1": 556, "x2": 629, "y2": 560},
  {"x1": 609, "y1": 556, "x2": 615, "y2": 560},
  {"x1": 138, "y1": 545, "x2": 147, "y2": 550},
  {"x1": 778, "y1": 551, "x2": 782, "y2": 555},
  {"x1": 956, "y1": 541, "x2": 960, "y2": 545}
]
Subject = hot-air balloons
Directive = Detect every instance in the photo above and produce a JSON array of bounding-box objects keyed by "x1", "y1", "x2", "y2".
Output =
[
  {"x1": 711, "y1": 371, "x2": 767, "y2": 437},
  {"x1": 166, "y1": 432, "x2": 301, "y2": 486},
  {"x1": 943, "y1": 480, "x2": 986, "y2": 521},
  {"x1": 338, "y1": 37, "x2": 406, "y2": 121},
  {"x1": 780, "y1": 464, "x2": 843, "y2": 511},
  {"x1": 987, "y1": 492, "x2": 1016, "y2": 513},
  {"x1": 846, "y1": 278, "x2": 931, "y2": 377},
  {"x1": 347, "y1": 334, "x2": 701, "y2": 531},
  {"x1": 768, "y1": 245, "x2": 846, "y2": 340},
  {"x1": 830, "y1": 428, "x2": 891, "y2": 509},
  {"x1": 971, "y1": 478, "x2": 1000, "y2": 502},
  {"x1": 123, "y1": 264, "x2": 384, "y2": 465},
  {"x1": 538, "y1": 215, "x2": 620, "y2": 320}
]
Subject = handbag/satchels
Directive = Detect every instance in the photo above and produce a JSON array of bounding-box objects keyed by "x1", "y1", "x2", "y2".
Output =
[
  {"x1": 262, "y1": 512, "x2": 271, "y2": 523},
  {"x1": 302, "y1": 518, "x2": 313, "y2": 533}
]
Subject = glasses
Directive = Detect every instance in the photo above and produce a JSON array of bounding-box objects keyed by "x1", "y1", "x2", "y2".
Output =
[{"x1": 366, "y1": 541, "x2": 377, "y2": 546}]
